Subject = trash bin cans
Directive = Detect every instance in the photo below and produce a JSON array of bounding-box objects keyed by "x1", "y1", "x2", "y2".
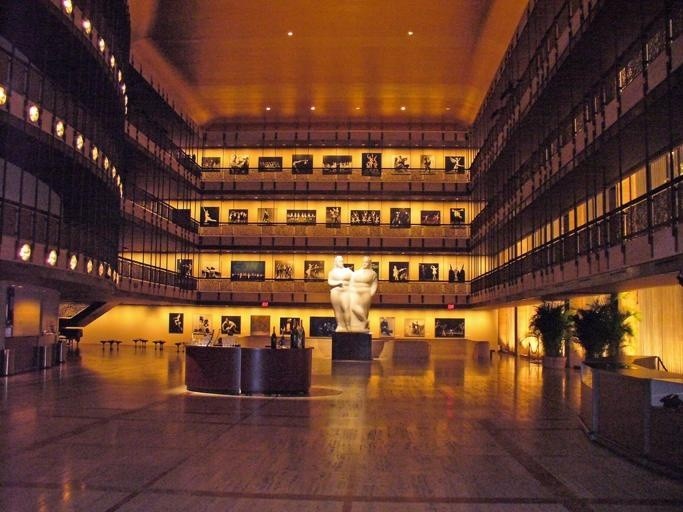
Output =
[
  {"x1": 43, "y1": 346, "x2": 50, "y2": 367},
  {"x1": 1, "y1": 348, "x2": 15, "y2": 376},
  {"x1": 59, "y1": 341, "x2": 65, "y2": 362}
]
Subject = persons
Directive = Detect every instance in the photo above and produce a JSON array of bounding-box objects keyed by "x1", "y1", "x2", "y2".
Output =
[
  {"x1": 202, "y1": 207, "x2": 465, "y2": 228},
  {"x1": 167, "y1": 313, "x2": 335, "y2": 335},
  {"x1": 381, "y1": 317, "x2": 465, "y2": 339},
  {"x1": 178, "y1": 261, "x2": 322, "y2": 281},
  {"x1": 327, "y1": 255, "x2": 353, "y2": 333},
  {"x1": 390, "y1": 263, "x2": 438, "y2": 283},
  {"x1": 202, "y1": 153, "x2": 466, "y2": 176},
  {"x1": 348, "y1": 255, "x2": 378, "y2": 334}
]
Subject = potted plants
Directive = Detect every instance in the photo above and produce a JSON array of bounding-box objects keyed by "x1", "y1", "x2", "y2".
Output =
[{"x1": 528, "y1": 299, "x2": 576, "y2": 370}]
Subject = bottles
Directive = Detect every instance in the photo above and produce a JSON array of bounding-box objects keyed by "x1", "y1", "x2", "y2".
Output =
[
  {"x1": 270, "y1": 326, "x2": 276, "y2": 350},
  {"x1": 290, "y1": 318, "x2": 304, "y2": 348}
]
